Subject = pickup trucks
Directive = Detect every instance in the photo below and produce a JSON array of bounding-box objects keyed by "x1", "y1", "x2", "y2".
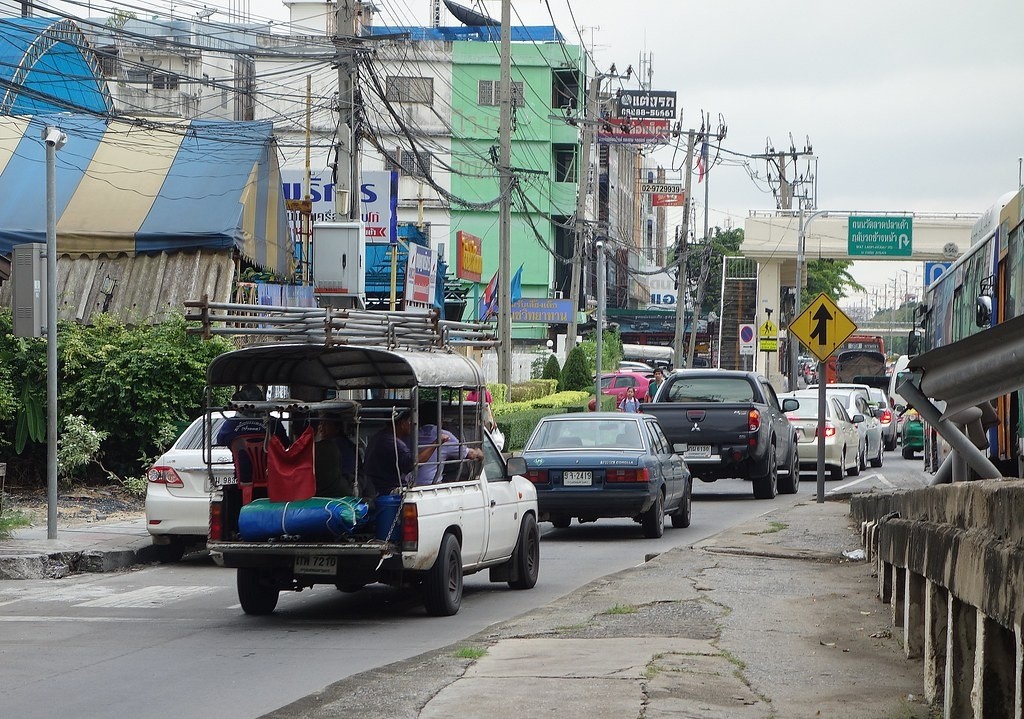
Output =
[
  {"x1": 637, "y1": 368, "x2": 800, "y2": 499},
  {"x1": 204, "y1": 341, "x2": 543, "y2": 616}
]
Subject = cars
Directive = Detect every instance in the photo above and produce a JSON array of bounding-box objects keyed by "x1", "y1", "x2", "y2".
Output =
[
  {"x1": 790, "y1": 350, "x2": 925, "y2": 471},
  {"x1": 776, "y1": 393, "x2": 861, "y2": 481},
  {"x1": 143, "y1": 410, "x2": 290, "y2": 566},
  {"x1": 521, "y1": 412, "x2": 694, "y2": 539}
]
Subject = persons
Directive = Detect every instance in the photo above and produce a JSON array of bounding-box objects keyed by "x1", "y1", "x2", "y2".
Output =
[
  {"x1": 644, "y1": 368, "x2": 665, "y2": 403},
  {"x1": 784, "y1": 371, "x2": 789, "y2": 393},
  {"x1": 619, "y1": 387, "x2": 640, "y2": 414},
  {"x1": 216, "y1": 384, "x2": 497, "y2": 512}
]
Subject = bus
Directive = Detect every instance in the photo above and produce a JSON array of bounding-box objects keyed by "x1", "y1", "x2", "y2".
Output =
[
  {"x1": 826, "y1": 335, "x2": 885, "y2": 386},
  {"x1": 998, "y1": 187, "x2": 1024, "y2": 479},
  {"x1": 907, "y1": 224, "x2": 1001, "y2": 477}
]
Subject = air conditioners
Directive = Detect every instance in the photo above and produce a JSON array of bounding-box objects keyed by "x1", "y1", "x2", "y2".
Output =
[{"x1": 555, "y1": 291, "x2": 563, "y2": 301}]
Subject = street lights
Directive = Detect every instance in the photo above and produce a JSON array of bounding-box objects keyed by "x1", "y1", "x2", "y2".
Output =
[{"x1": 791, "y1": 208, "x2": 828, "y2": 395}]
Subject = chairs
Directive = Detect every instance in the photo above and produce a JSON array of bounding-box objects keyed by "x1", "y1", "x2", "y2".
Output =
[{"x1": 231, "y1": 432, "x2": 269, "y2": 508}]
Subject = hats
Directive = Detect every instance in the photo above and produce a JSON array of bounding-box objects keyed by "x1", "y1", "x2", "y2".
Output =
[{"x1": 654, "y1": 368, "x2": 663, "y2": 374}]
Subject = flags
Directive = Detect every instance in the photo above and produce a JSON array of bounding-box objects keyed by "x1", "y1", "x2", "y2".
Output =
[{"x1": 484, "y1": 266, "x2": 523, "y2": 312}]
